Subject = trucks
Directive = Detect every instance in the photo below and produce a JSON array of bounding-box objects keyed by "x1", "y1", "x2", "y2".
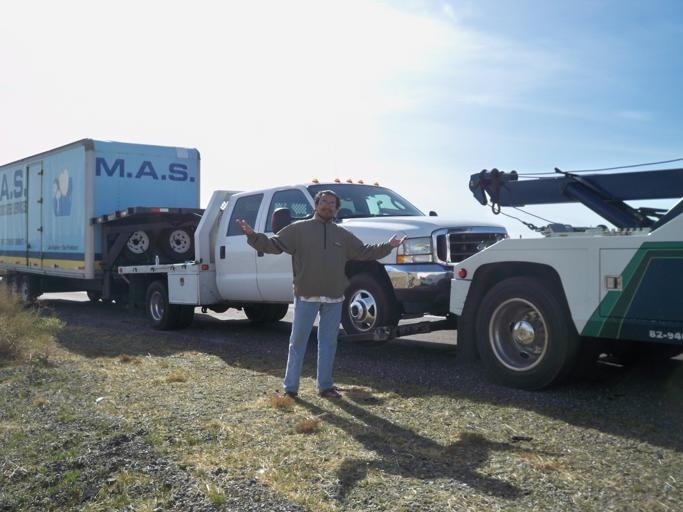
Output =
[{"x1": 0, "y1": 137, "x2": 512, "y2": 344}]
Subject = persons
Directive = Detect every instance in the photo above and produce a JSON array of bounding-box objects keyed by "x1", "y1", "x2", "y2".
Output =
[{"x1": 233, "y1": 189, "x2": 407, "y2": 400}]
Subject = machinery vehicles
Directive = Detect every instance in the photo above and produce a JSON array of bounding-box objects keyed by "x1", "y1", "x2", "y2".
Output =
[{"x1": 448, "y1": 146, "x2": 682, "y2": 391}]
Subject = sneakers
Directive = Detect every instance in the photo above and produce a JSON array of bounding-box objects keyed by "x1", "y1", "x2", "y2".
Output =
[{"x1": 319, "y1": 389, "x2": 338, "y2": 398}]
